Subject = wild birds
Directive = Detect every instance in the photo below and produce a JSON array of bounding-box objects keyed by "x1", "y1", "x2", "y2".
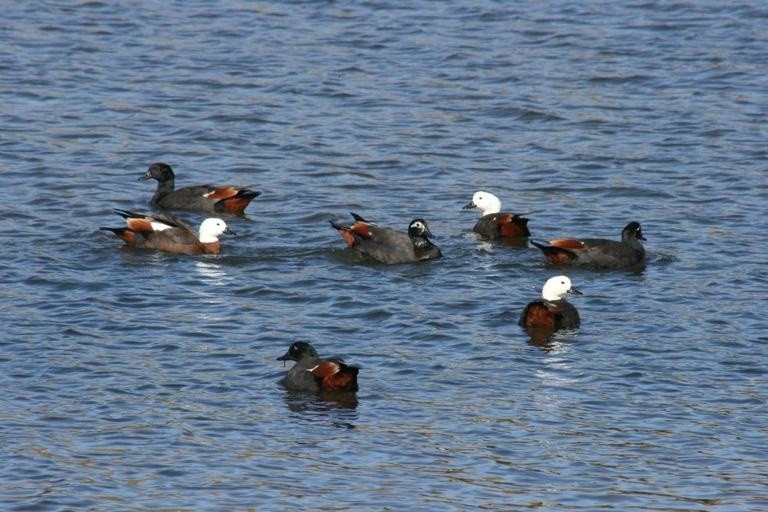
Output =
[
  {"x1": 97, "y1": 206, "x2": 238, "y2": 257},
  {"x1": 529, "y1": 220, "x2": 649, "y2": 269},
  {"x1": 136, "y1": 161, "x2": 263, "y2": 215},
  {"x1": 461, "y1": 191, "x2": 531, "y2": 238},
  {"x1": 516, "y1": 274, "x2": 584, "y2": 329},
  {"x1": 328, "y1": 210, "x2": 444, "y2": 266},
  {"x1": 274, "y1": 339, "x2": 362, "y2": 393}
]
